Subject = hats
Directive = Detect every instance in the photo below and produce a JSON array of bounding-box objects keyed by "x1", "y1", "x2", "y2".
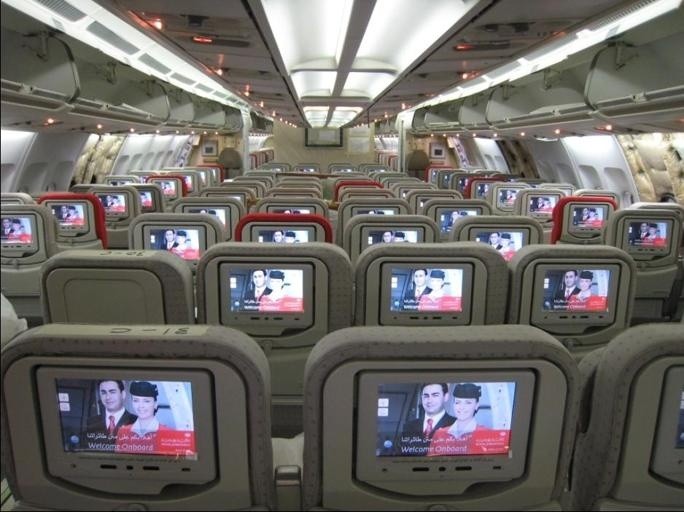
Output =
[
  {"x1": 453, "y1": 384, "x2": 480, "y2": 398},
  {"x1": 285, "y1": 232, "x2": 295, "y2": 237},
  {"x1": 130, "y1": 382, "x2": 157, "y2": 397},
  {"x1": 431, "y1": 271, "x2": 444, "y2": 278},
  {"x1": 177, "y1": 231, "x2": 186, "y2": 236},
  {"x1": 270, "y1": 271, "x2": 283, "y2": 279},
  {"x1": 650, "y1": 224, "x2": 657, "y2": 227},
  {"x1": 13, "y1": 220, "x2": 20, "y2": 223},
  {"x1": 501, "y1": 234, "x2": 510, "y2": 238},
  {"x1": 580, "y1": 271, "x2": 593, "y2": 279},
  {"x1": 395, "y1": 233, "x2": 404, "y2": 237},
  {"x1": 69, "y1": 206, "x2": 74, "y2": 209}
]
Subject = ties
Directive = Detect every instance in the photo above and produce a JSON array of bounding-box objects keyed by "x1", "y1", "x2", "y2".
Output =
[
  {"x1": 426, "y1": 420, "x2": 433, "y2": 436},
  {"x1": 567, "y1": 290, "x2": 569, "y2": 298},
  {"x1": 108, "y1": 415, "x2": 115, "y2": 434}
]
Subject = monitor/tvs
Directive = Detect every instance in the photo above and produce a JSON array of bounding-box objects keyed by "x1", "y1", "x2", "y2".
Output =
[
  {"x1": 379, "y1": 261, "x2": 474, "y2": 326},
  {"x1": 107, "y1": 168, "x2": 218, "y2": 193},
  {"x1": 403, "y1": 168, "x2": 572, "y2": 213},
  {"x1": 47, "y1": 202, "x2": 90, "y2": 237},
  {"x1": 267, "y1": 205, "x2": 316, "y2": 216},
  {"x1": 94, "y1": 192, "x2": 129, "y2": 222},
  {"x1": 135, "y1": 188, "x2": 157, "y2": 213},
  {"x1": 251, "y1": 226, "x2": 316, "y2": 243},
  {"x1": 622, "y1": 218, "x2": 675, "y2": 261},
  {"x1": 265, "y1": 166, "x2": 387, "y2": 174},
  {"x1": 353, "y1": 367, "x2": 536, "y2": 500},
  {"x1": 150, "y1": 179, "x2": 178, "y2": 202},
  {"x1": 360, "y1": 225, "x2": 425, "y2": 256},
  {"x1": 0, "y1": 214, "x2": 39, "y2": 258},
  {"x1": 568, "y1": 204, "x2": 609, "y2": 238},
  {"x1": 218, "y1": 261, "x2": 314, "y2": 337},
  {"x1": 35, "y1": 365, "x2": 218, "y2": 497},
  {"x1": 531, "y1": 261, "x2": 621, "y2": 336},
  {"x1": 469, "y1": 227, "x2": 530, "y2": 265},
  {"x1": 181, "y1": 193, "x2": 244, "y2": 240},
  {"x1": 351, "y1": 206, "x2": 400, "y2": 216},
  {"x1": 649, "y1": 363, "x2": 683, "y2": 489},
  {"x1": 435, "y1": 207, "x2": 482, "y2": 241},
  {"x1": 143, "y1": 225, "x2": 207, "y2": 273},
  {"x1": 526, "y1": 194, "x2": 560, "y2": 223}
]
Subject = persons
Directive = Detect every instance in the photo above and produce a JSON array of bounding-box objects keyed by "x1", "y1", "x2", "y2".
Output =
[
  {"x1": 1, "y1": 165, "x2": 669, "y2": 258},
  {"x1": 84, "y1": 380, "x2": 172, "y2": 454},
  {"x1": 555, "y1": 270, "x2": 597, "y2": 310},
  {"x1": 244, "y1": 269, "x2": 286, "y2": 311},
  {"x1": 403, "y1": 268, "x2": 447, "y2": 310},
  {"x1": 401, "y1": 383, "x2": 488, "y2": 455}
]
